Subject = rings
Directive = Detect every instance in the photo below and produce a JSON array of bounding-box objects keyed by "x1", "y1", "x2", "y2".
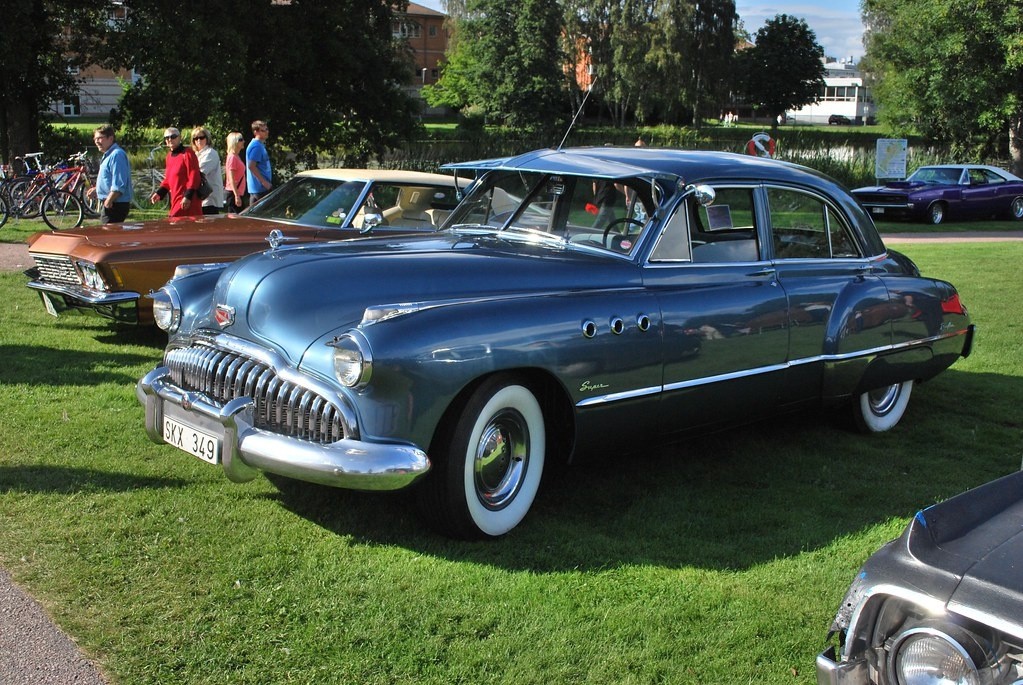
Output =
[
  {"x1": 188, "y1": 206, "x2": 190, "y2": 208},
  {"x1": 184, "y1": 205, "x2": 186, "y2": 207}
]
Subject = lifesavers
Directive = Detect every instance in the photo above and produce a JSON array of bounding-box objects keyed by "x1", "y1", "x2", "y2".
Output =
[{"x1": 748, "y1": 134, "x2": 777, "y2": 158}]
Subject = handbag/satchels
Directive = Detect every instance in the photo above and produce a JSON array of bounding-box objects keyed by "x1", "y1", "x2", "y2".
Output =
[
  {"x1": 222, "y1": 189, "x2": 235, "y2": 207},
  {"x1": 199, "y1": 171, "x2": 213, "y2": 199}
]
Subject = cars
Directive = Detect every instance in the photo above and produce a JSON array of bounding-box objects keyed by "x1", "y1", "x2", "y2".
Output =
[
  {"x1": 829, "y1": 114, "x2": 850, "y2": 126},
  {"x1": 816, "y1": 468, "x2": 1023, "y2": 685},
  {"x1": 135, "y1": 144, "x2": 977, "y2": 544},
  {"x1": 23, "y1": 168, "x2": 617, "y2": 326},
  {"x1": 848, "y1": 164, "x2": 1023, "y2": 226}
]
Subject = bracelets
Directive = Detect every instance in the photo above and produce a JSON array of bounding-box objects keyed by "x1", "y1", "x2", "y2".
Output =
[{"x1": 235, "y1": 197, "x2": 240, "y2": 199}]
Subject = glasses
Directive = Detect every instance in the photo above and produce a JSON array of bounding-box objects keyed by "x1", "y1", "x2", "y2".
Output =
[
  {"x1": 261, "y1": 128, "x2": 268, "y2": 132},
  {"x1": 163, "y1": 134, "x2": 179, "y2": 140},
  {"x1": 194, "y1": 135, "x2": 205, "y2": 140},
  {"x1": 238, "y1": 138, "x2": 244, "y2": 142}
]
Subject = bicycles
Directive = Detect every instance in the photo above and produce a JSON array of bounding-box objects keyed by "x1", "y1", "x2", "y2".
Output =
[
  {"x1": 0, "y1": 151, "x2": 102, "y2": 232},
  {"x1": 132, "y1": 146, "x2": 171, "y2": 211}
]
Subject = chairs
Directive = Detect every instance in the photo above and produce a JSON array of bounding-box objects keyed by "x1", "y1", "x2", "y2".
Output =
[{"x1": 390, "y1": 208, "x2": 439, "y2": 231}]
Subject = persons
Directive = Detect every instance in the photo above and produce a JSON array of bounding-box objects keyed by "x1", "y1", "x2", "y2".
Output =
[
  {"x1": 86, "y1": 125, "x2": 132, "y2": 226},
  {"x1": 591, "y1": 138, "x2": 650, "y2": 231},
  {"x1": 719, "y1": 109, "x2": 739, "y2": 128},
  {"x1": 777, "y1": 113, "x2": 783, "y2": 126},
  {"x1": 150, "y1": 127, "x2": 203, "y2": 218},
  {"x1": 190, "y1": 119, "x2": 273, "y2": 218}
]
